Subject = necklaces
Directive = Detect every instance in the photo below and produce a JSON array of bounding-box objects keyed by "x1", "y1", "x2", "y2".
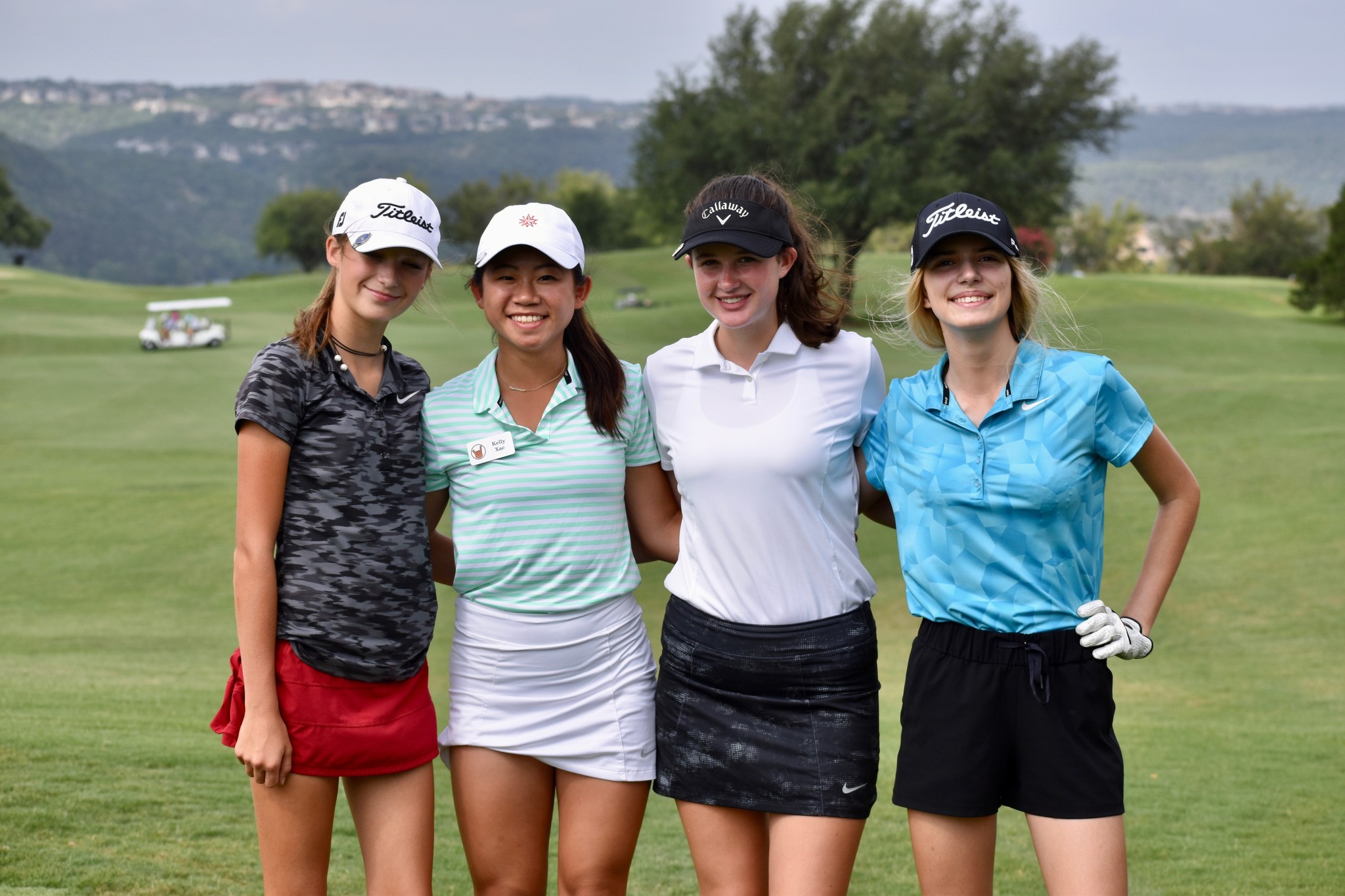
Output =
[
  {"x1": 321, "y1": 323, "x2": 387, "y2": 371},
  {"x1": 495, "y1": 362, "x2": 566, "y2": 392}
]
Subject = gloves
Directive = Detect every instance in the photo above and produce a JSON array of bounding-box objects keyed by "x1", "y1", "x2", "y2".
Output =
[{"x1": 1076, "y1": 600, "x2": 1152, "y2": 660}]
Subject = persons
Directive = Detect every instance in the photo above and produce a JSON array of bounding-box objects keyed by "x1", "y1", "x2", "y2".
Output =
[
  {"x1": 420, "y1": 203, "x2": 682, "y2": 896},
  {"x1": 639, "y1": 172, "x2": 888, "y2": 896},
  {"x1": 854, "y1": 193, "x2": 1199, "y2": 896},
  {"x1": 209, "y1": 177, "x2": 455, "y2": 896}
]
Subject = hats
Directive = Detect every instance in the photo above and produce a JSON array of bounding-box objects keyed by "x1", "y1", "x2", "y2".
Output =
[
  {"x1": 332, "y1": 178, "x2": 443, "y2": 270},
  {"x1": 474, "y1": 202, "x2": 585, "y2": 275},
  {"x1": 910, "y1": 191, "x2": 1021, "y2": 273},
  {"x1": 672, "y1": 199, "x2": 794, "y2": 260}
]
